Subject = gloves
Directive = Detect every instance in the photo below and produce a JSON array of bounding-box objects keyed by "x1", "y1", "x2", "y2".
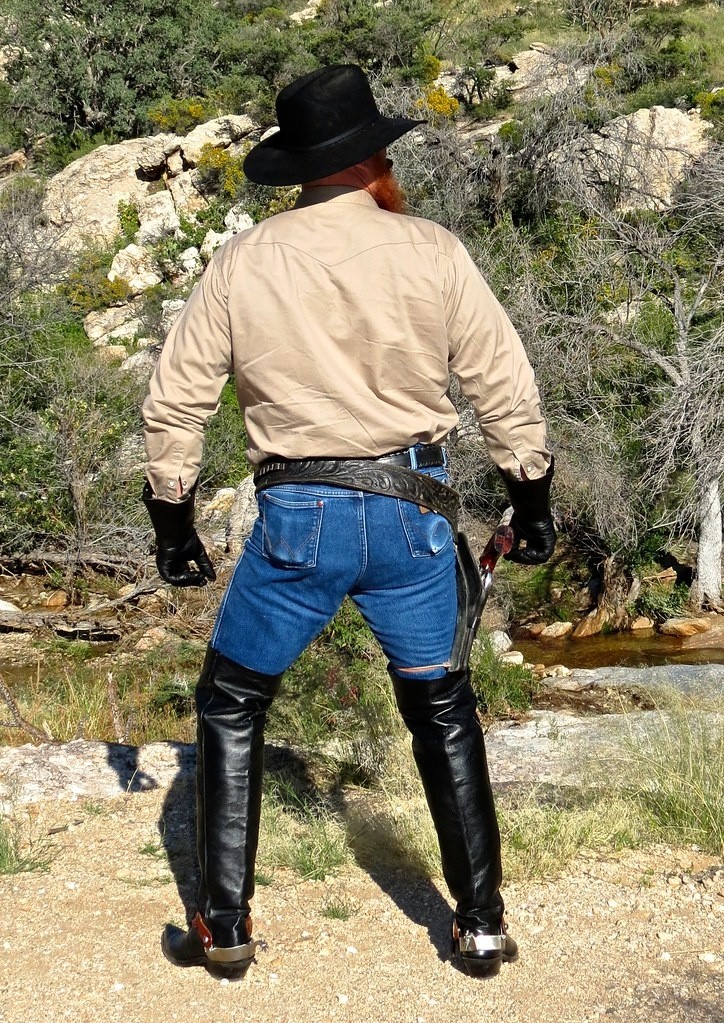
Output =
[
  {"x1": 497, "y1": 455, "x2": 557, "y2": 565},
  {"x1": 142, "y1": 476, "x2": 218, "y2": 587}
]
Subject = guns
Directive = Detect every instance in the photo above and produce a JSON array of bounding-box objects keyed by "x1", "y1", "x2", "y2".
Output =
[{"x1": 477, "y1": 526, "x2": 514, "y2": 583}]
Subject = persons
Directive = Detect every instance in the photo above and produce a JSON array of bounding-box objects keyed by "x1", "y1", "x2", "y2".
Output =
[{"x1": 140, "y1": 62, "x2": 558, "y2": 984}]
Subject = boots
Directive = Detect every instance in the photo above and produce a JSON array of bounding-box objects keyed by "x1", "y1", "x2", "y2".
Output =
[
  {"x1": 386, "y1": 662, "x2": 519, "y2": 980},
  {"x1": 161, "y1": 642, "x2": 285, "y2": 981}
]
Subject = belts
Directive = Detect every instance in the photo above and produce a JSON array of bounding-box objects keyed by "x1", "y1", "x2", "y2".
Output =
[
  {"x1": 253, "y1": 458, "x2": 461, "y2": 538},
  {"x1": 373, "y1": 446, "x2": 444, "y2": 469}
]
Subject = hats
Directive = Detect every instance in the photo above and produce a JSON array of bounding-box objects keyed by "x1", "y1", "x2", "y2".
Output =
[{"x1": 243, "y1": 64, "x2": 428, "y2": 186}]
